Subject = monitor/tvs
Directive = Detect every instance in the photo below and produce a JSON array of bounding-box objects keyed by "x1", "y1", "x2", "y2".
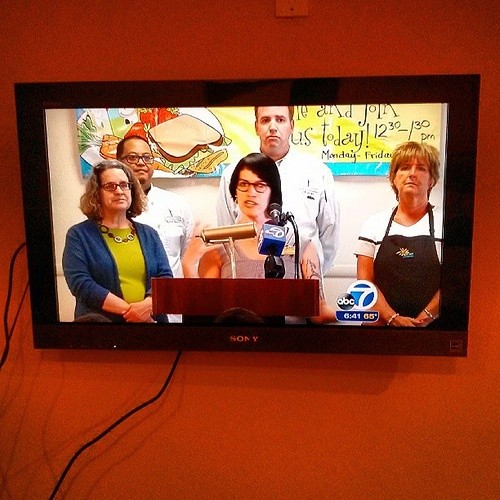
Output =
[{"x1": 12, "y1": 70, "x2": 485, "y2": 355}]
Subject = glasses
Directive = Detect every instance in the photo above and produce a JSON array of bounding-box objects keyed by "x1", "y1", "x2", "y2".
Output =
[
  {"x1": 99, "y1": 182, "x2": 134, "y2": 191},
  {"x1": 235, "y1": 178, "x2": 269, "y2": 193},
  {"x1": 119, "y1": 154, "x2": 155, "y2": 164}
]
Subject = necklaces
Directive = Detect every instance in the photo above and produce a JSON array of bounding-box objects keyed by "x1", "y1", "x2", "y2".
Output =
[{"x1": 98, "y1": 220, "x2": 136, "y2": 243}]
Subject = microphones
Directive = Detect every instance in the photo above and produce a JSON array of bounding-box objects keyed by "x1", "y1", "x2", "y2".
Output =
[
  {"x1": 266, "y1": 202, "x2": 284, "y2": 226},
  {"x1": 199, "y1": 222, "x2": 257, "y2": 243},
  {"x1": 264, "y1": 218, "x2": 278, "y2": 260}
]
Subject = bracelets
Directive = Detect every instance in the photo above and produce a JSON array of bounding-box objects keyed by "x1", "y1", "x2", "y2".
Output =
[
  {"x1": 424, "y1": 309, "x2": 436, "y2": 319},
  {"x1": 387, "y1": 313, "x2": 399, "y2": 325}
]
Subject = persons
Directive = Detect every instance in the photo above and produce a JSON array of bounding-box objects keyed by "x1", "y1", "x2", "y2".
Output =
[
  {"x1": 198, "y1": 152, "x2": 336, "y2": 324},
  {"x1": 116, "y1": 134, "x2": 194, "y2": 324},
  {"x1": 62, "y1": 159, "x2": 174, "y2": 324},
  {"x1": 353, "y1": 140, "x2": 444, "y2": 327},
  {"x1": 217, "y1": 106, "x2": 337, "y2": 276}
]
